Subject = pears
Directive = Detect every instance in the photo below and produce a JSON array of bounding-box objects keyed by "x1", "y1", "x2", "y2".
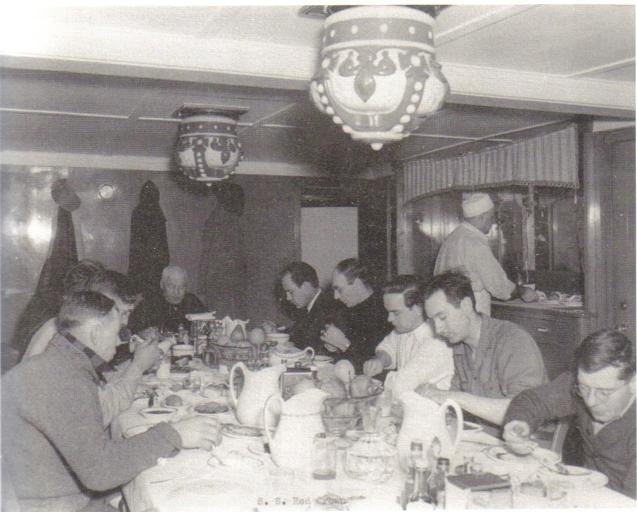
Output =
[{"x1": 230, "y1": 324, "x2": 244, "y2": 342}]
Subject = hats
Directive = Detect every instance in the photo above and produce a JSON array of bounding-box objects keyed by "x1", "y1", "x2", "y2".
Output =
[{"x1": 461, "y1": 191, "x2": 494, "y2": 219}]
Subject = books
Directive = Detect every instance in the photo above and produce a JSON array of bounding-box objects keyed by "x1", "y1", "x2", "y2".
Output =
[{"x1": 448, "y1": 471, "x2": 513, "y2": 492}]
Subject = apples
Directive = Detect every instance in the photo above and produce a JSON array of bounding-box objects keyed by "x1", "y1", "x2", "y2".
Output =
[
  {"x1": 334, "y1": 359, "x2": 355, "y2": 383},
  {"x1": 350, "y1": 373, "x2": 373, "y2": 397},
  {"x1": 249, "y1": 328, "x2": 265, "y2": 345}
]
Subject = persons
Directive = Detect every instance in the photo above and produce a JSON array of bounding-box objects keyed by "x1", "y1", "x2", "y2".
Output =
[
  {"x1": 362, "y1": 272, "x2": 455, "y2": 407},
  {"x1": 433, "y1": 193, "x2": 539, "y2": 318},
  {"x1": 1, "y1": 289, "x2": 223, "y2": 512},
  {"x1": 279, "y1": 262, "x2": 332, "y2": 355},
  {"x1": 414, "y1": 264, "x2": 550, "y2": 439},
  {"x1": 19, "y1": 260, "x2": 164, "y2": 428},
  {"x1": 128, "y1": 265, "x2": 209, "y2": 337},
  {"x1": 502, "y1": 326, "x2": 636, "y2": 501},
  {"x1": 318, "y1": 255, "x2": 393, "y2": 377}
]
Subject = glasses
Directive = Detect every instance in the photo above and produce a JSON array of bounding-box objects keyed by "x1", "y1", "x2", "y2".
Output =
[{"x1": 571, "y1": 379, "x2": 628, "y2": 403}]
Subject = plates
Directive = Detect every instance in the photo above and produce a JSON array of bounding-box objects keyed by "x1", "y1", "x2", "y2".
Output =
[{"x1": 110, "y1": 312, "x2": 610, "y2": 512}]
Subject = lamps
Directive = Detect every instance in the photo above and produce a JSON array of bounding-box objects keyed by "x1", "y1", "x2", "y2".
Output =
[
  {"x1": 171, "y1": 104, "x2": 249, "y2": 187},
  {"x1": 295, "y1": 5, "x2": 450, "y2": 150}
]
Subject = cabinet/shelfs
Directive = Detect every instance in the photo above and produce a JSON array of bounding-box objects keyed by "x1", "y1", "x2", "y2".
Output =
[{"x1": 492, "y1": 304, "x2": 584, "y2": 382}]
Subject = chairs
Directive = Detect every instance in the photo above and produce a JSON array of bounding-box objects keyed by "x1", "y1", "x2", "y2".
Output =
[{"x1": 534, "y1": 417, "x2": 571, "y2": 454}]
